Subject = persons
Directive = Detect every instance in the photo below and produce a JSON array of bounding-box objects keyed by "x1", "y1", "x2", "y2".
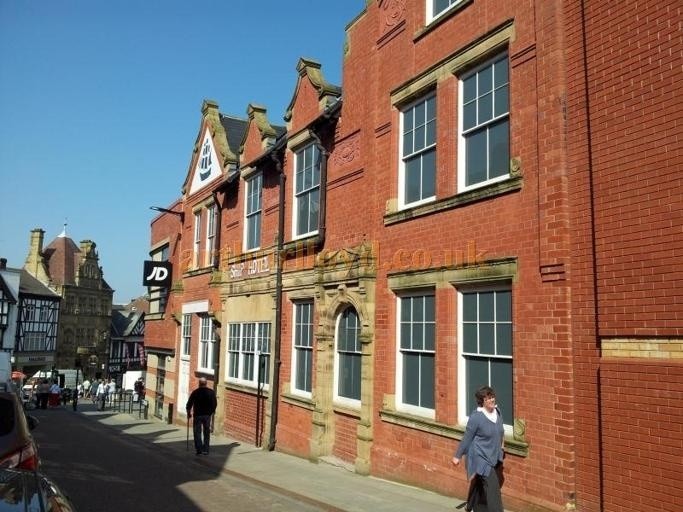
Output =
[
  {"x1": 77, "y1": 377, "x2": 117, "y2": 411},
  {"x1": 40, "y1": 379, "x2": 51, "y2": 409},
  {"x1": 134, "y1": 377, "x2": 145, "y2": 403},
  {"x1": 450, "y1": 385, "x2": 506, "y2": 512},
  {"x1": 35, "y1": 380, "x2": 43, "y2": 409},
  {"x1": 186, "y1": 376, "x2": 217, "y2": 456},
  {"x1": 48, "y1": 380, "x2": 62, "y2": 408}
]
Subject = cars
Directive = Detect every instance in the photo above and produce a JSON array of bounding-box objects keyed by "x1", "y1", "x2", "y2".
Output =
[
  {"x1": 0, "y1": 391, "x2": 42, "y2": 473},
  {"x1": 0, "y1": 467, "x2": 79, "y2": 512}
]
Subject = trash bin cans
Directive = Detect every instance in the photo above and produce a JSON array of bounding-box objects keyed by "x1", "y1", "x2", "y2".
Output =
[{"x1": 168, "y1": 403, "x2": 173, "y2": 424}]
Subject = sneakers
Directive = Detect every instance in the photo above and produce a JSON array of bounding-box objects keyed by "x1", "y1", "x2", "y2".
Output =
[{"x1": 195, "y1": 451, "x2": 209, "y2": 456}]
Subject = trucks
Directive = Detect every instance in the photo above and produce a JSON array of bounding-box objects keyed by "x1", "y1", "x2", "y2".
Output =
[{"x1": 19, "y1": 369, "x2": 83, "y2": 408}]
